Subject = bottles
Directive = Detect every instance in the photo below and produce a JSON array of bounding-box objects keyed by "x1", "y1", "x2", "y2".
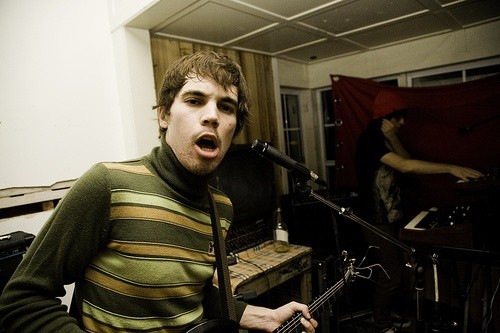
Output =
[{"x1": 273, "y1": 208, "x2": 289, "y2": 253}]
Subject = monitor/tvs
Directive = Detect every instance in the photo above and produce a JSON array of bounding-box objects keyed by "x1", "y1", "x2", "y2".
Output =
[{"x1": 208, "y1": 143, "x2": 277, "y2": 228}]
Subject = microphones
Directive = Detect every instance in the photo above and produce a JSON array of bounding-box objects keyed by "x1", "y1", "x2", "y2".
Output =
[{"x1": 250, "y1": 139, "x2": 327, "y2": 186}]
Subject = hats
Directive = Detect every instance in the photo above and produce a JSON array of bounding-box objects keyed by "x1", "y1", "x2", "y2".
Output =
[{"x1": 369, "y1": 84, "x2": 411, "y2": 119}]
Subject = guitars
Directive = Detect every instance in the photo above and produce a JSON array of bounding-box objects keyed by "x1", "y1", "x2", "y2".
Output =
[{"x1": 185, "y1": 245, "x2": 390, "y2": 333}]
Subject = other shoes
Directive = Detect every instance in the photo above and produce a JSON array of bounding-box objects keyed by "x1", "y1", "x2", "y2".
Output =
[
  {"x1": 390, "y1": 314, "x2": 411, "y2": 328},
  {"x1": 382, "y1": 326, "x2": 397, "y2": 333}
]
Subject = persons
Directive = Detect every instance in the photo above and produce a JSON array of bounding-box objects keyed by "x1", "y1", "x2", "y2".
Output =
[
  {"x1": 352, "y1": 90, "x2": 484, "y2": 333},
  {"x1": 1, "y1": 48, "x2": 322, "y2": 333}
]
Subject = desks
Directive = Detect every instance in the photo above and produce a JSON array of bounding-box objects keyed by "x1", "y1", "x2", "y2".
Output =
[{"x1": 212, "y1": 241, "x2": 314, "y2": 305}]
